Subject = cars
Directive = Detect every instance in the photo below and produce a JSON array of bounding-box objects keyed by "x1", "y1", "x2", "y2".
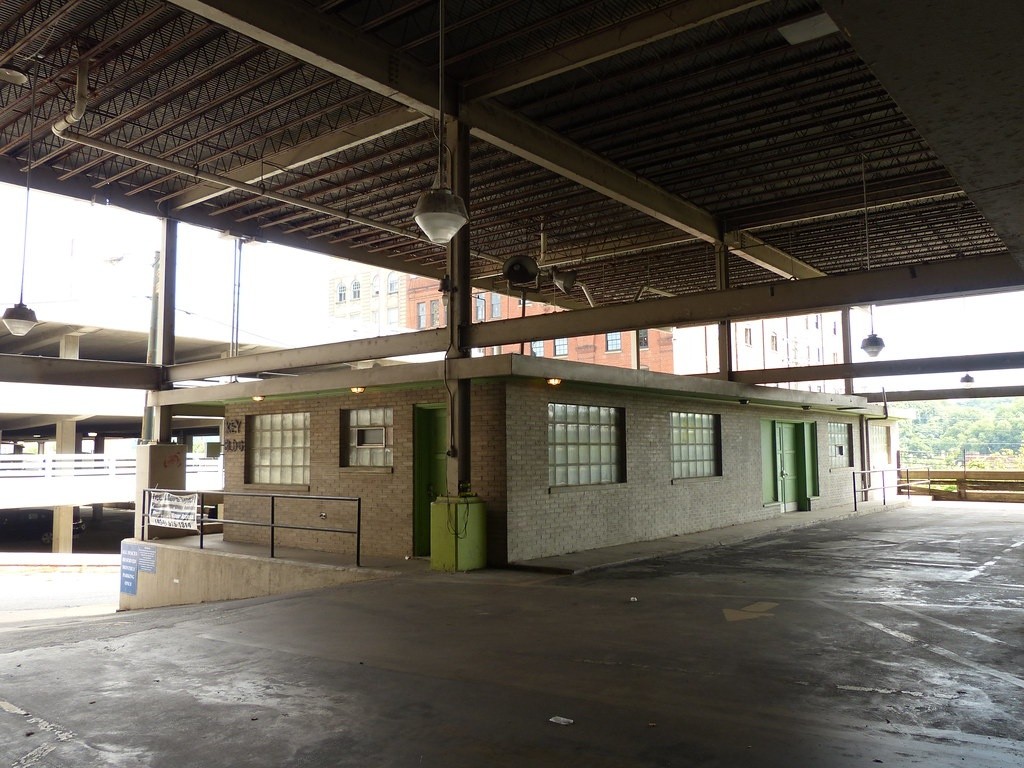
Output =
[{"x1": 0, "y1": 510, "x2": 86, "y2": 546}]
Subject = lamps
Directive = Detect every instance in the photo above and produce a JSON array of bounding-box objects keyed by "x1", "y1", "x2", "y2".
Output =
[
  {"x1": 0, "y1": 55, "x2": 40, "y2": 337},
  {"x1": 351, "y1": 387, "x2": 366, "y2": 394},
  {"x1": 860, "y1": 155, "x2": 885, "y2": 358},
  {"x1": 544, "y1": 378, "x2": 564, "y2": 387},
  {"x1": 739, "y1": 400, "x2": 751, "y2": 404},
  {"x1": 960, "y1": 371, "x2": 975, "y2": 383},
  {"x1": 802, "y1": 406, "x2": 811, "y2": 410},
  {"x1": 252, "y1": 396, "x2": 266, "y2": 401},
  {"x1": 411, "y1": 0, "x2": 472, "y2": 247},
  {"x1": 0, "y1": 58, "x2": 30, "y2": 86}
]
